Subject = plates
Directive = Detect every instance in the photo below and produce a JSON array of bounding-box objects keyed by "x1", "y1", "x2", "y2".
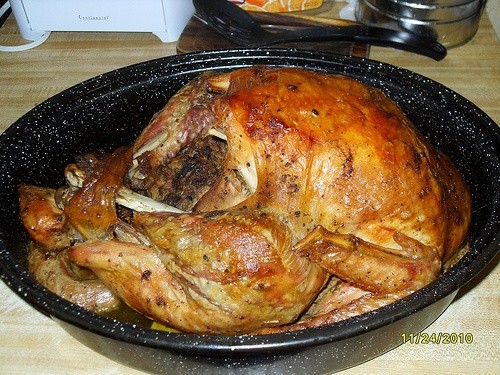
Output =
[{"x1": 0, "y1": 47, "x2": 500, "y2": 354}]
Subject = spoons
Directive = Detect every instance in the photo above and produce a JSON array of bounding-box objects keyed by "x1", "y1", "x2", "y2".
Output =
[{"x1": 193, "y1": 0, "x2": 447, "y2": 61}]
